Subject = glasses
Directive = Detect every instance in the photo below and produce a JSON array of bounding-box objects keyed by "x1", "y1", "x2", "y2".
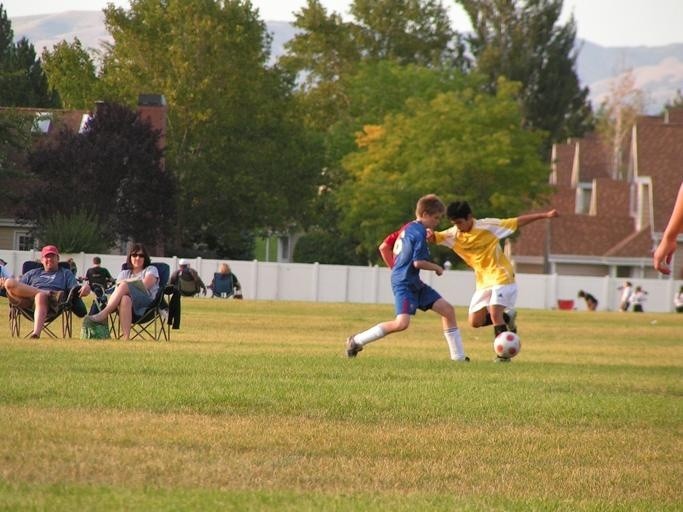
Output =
[{"x1": 130, "y1": 253, "x2": 144, "y2": 257}]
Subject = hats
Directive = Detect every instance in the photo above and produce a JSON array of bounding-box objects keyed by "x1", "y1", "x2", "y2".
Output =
[
  {"x1": 40, "y1": 245, "x2": 59, "y2": 258},
  {"x1": 178, "y1": 258, "x2": 189, "y2": 265}
]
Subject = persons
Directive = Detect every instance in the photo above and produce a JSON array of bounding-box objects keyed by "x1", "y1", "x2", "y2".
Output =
[
  {"x1": 619, "y1": 281, "x2": 633, "y2": 312},
  {"x1": 578, "y1": 289, "x2": 598, "y2": 311},
  {"x1": 0, "y1": 242, "x2": 244, "y2": 341},
  {"x1": 347, "y1": 194, "x2": 470, "y2": 362},
  {"x1": 654, "y1": 182, "x2": 683, "y2": 275},
  {"x1": 633, "y1": 286, "x2": 648, "y2": 312},
  {"x1": 673, "y1": 284, "x2": 683, "y2": 313},
  {"x1": 426, "y1": 200, "x2": 559, "y2": 362}
]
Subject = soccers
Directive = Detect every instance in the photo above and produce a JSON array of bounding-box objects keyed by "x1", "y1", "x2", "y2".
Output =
[{"x1": 494, "y1": 331, "x2": 521, "y2": 357}]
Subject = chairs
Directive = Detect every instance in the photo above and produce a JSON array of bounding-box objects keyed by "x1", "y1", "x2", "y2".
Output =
[
  {"x1": 88, "y1": 275, "x2": 115, "y2": 293},
  {"x1": 7, "y1": 261, "x2": 77, "y2": 338},
  {"x1": 170, "y1": 270, "x2": 202, "y2": 298},
  {"x1": 206, "y1": 271, "x2": 237, "y2": 299},
  {"x1": 93, "y1": 261, "x2": 177, "y2": 343}
]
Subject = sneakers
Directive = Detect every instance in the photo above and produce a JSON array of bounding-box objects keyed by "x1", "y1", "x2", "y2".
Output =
[
  {"x1": 88, "y1": 315, "x2": 107, "y2": 325},
  {"x1": 450, "y1": 356, "x2": 470, "y2": 363},
  {"x1": 47, "y1": 289, "x2": 64, "y2": 305},
  {"x1": 504, "y1": 308, "x2": 518, "y2": 334},
  {"x1": 27, "y1": 334, "x2": 41, "y2": 340},
  {"x1": 345, "y1": 334, "x2": 363, "y2": 360},
  {"x1": 490, "y1": 354, "x2": 511, "y2": 364}
]
